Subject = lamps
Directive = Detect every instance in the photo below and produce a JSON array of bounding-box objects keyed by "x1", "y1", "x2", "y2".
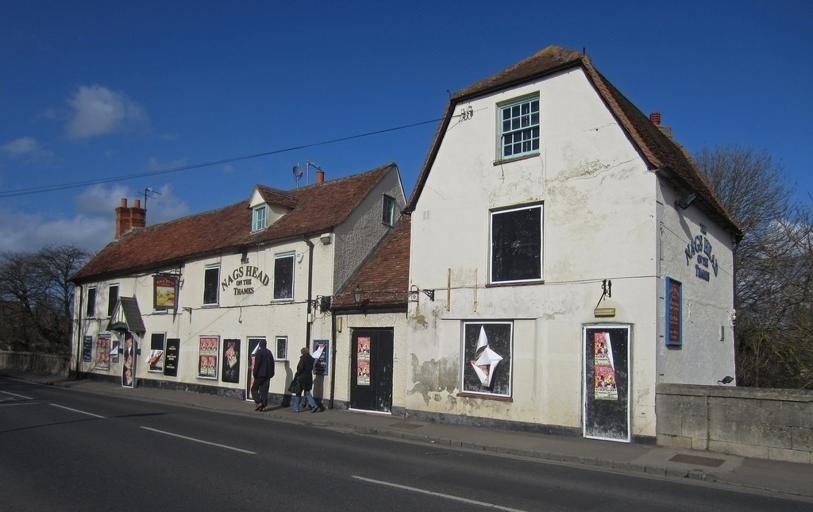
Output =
[
  {"x1": 405, "y1": 283, "x2": 435, "y2": 303},
  {"x1": 352, "y1": 282, "x2": 369, "y2": 310}
]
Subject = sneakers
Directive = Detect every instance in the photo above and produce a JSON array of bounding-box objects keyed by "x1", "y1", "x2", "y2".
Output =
[{"x1": 310, "y1": 404, "x2": 323, "y2": 413}]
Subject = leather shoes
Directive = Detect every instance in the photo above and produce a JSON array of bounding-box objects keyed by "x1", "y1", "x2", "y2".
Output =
[{"x1": 255, "y1": 404, "x2": 266, "y2": 411}]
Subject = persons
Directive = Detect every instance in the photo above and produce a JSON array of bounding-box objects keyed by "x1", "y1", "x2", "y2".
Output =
[
  {"x1": 251, "y1": 339, "x2": 275, "y2": 411},
  {"x1": 123, "y1": 334, "x2": 133, "y2": 386},
  {"x1": 288, "y1": 347, "x2": 320, "y2": 413}
]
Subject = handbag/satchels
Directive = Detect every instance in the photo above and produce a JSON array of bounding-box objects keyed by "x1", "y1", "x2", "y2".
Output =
[{"x1": 288, "y1": 381, "x2": 302, "y2": 396}]
